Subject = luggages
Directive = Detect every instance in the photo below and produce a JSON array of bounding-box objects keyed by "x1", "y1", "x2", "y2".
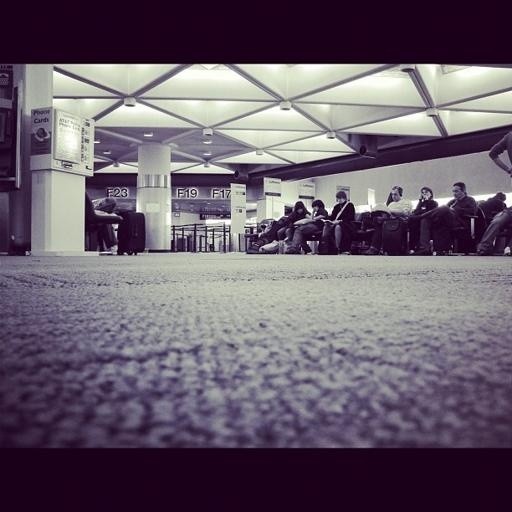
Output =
[
  {"x1": 117, "y1": 213, "x2": 145, "y2": 255},
  {"x1": 382, "y1": 220, "x2": 407, "y2": 256}
]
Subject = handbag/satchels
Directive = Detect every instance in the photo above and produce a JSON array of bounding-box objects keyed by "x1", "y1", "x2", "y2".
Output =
[{"x1": 350, "y1": 240, "x2": 368, "y2": 254}]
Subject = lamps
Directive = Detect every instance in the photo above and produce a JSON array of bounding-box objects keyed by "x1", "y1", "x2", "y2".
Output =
[
  {"x1": 426, "y1": 108, "x2": 438, "y2": 116},
  {"x1": 399, "y1": 64, "x2": 415, "y2": 72},
  {"x1": 94, "y1": 97, "x2": 335, "y2": 168}
]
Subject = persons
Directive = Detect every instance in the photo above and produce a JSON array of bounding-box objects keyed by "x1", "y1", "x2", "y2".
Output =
[
  {"x1": 489, "y1": 131, "x2": 512, "y2": 180},
  {"x1": 251, "y1": 181, "x2": 511, "y2": 257}
]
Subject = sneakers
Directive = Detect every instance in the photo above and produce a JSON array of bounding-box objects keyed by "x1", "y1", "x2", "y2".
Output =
[{"x1": 364, "y1": 248, "x2": 378, "y2": 255}]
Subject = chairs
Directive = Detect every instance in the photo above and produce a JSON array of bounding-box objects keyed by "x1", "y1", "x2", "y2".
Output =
[
  {"x1": 243, "y1": 210, "x2": 512, "y2": 255},
  {"x1": 85, "y1": 192, "x2": 123, "y2": 256}
]
